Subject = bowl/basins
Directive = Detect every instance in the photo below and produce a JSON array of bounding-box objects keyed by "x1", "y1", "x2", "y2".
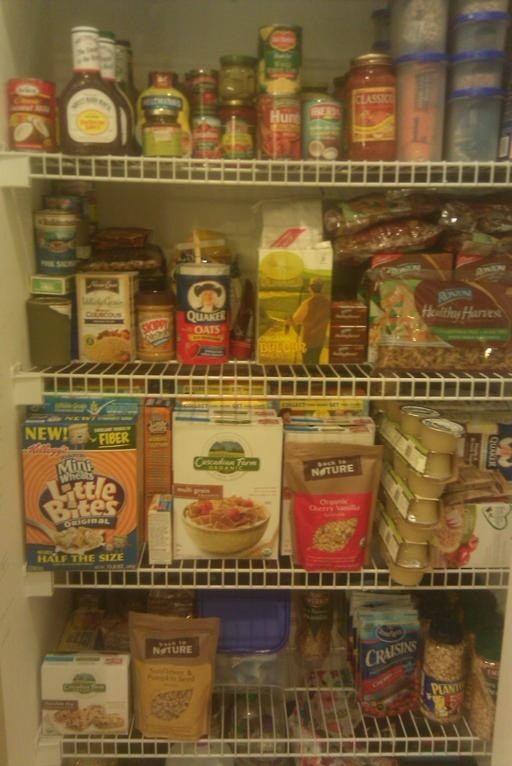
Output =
[{"x1": 179, "y1": 499, "x2": 270, "y2": 555}]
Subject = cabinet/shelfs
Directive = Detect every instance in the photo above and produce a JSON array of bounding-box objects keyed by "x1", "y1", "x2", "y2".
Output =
[{"x1": 0, "y1": 1, "x2": 511, "y2": 765}]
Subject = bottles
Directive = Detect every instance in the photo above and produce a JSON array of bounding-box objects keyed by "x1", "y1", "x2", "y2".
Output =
[
  {"x1": 57, "y1": 26, "x2": 136, "y2": 157},
  {"x1": 134, "y1": 277, "x2": 175, "y2": 362},
  {"x1": 298, "y1": 590, "x2": 332, "y2": 667},
  {"x1": 134, "y1": 70, "x2": 195, "y2": 160},
  {"x1": 372, "y1": 5, "x2": 395, "y2": 59},
  {"x1": 421, "y1": 593, "x2": 502, "y2": 740}
]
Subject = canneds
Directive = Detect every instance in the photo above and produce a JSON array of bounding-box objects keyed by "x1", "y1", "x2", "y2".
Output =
[
  {"x1": 7, "y1": 76, "x2": 57, "y2": 153},
  {"x1": 34, "y1": 208, "x2": 83, "y2": 274},
  {"x1": 138, "y1": 21, "x2": 397, "y2": 165},
  {"x1": 43, "y1": 193, "x2": 91, "y2": 259},
  {"x1": 134, "y1": 304, "x2": 175, "y2": 362},
  {"x1": 415, "y1": 576, "x2": 510, "y2": 744}
]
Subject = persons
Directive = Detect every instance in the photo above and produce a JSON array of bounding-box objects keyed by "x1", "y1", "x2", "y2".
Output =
[
  {"x1": 494, "y1": 434, "x2": 511, "y2": 476},
  {"x1": 291, "y1": 275, "x2": 333, "y2": 362},
  {"x1": 193, "y1": 282, "x2": 223, "y2": 314}
]
